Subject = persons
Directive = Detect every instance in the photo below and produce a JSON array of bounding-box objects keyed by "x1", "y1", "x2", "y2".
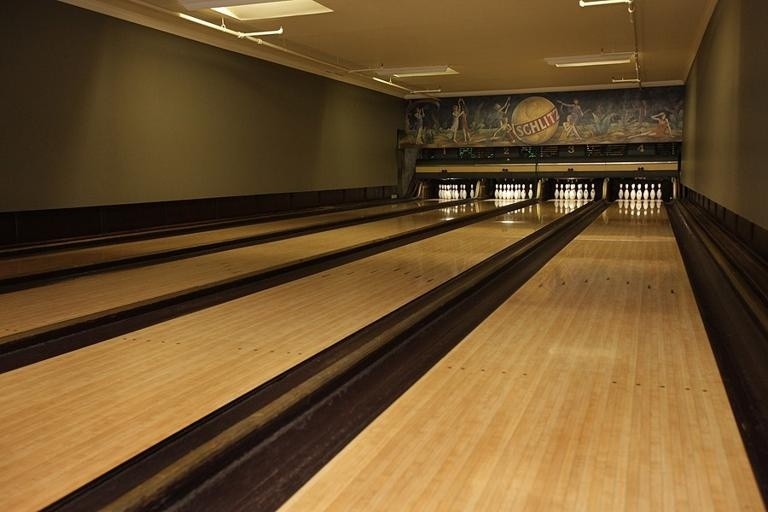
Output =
[{"x1": 408, "y1": 95, "x2": 683, "y2": 141}]
